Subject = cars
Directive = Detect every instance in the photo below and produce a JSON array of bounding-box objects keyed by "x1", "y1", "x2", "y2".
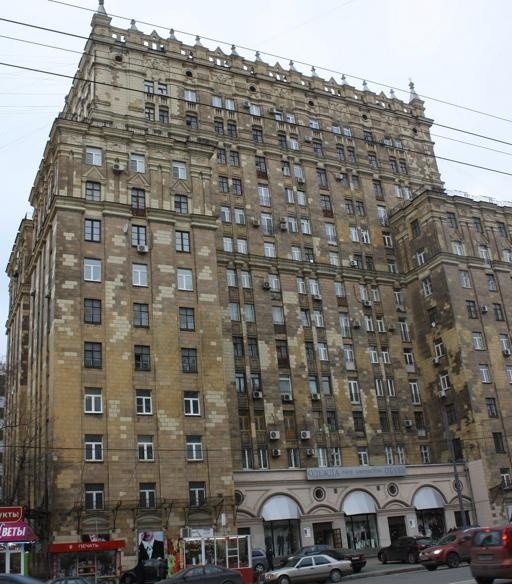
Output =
[
  {"x1": 417, "y1": 527, "x2": 475, "y2": 571},
  {"x1": 154, "y1": 565, "x2": 244, "y2": 584},
  {"x1": 252, "y1": 544, "x2": 367, "y2": 584},
  {"x1": 118, "y1": 560, "x2": 168, "y2": 584},
  {"x1": 378, "y1": 536, "x2": 437, "y2": 564}
]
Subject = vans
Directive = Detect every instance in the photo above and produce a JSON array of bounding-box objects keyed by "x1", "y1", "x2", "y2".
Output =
[{"x1": 470, "y1": 525, "x2": 512, "y2": 584}]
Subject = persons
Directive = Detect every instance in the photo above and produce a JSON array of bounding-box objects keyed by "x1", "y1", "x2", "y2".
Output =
[
  {"x1": 347, "y1": 526, "x2": 367, "y2": 547},
  {"x1": 139, "y1": 531, "x2": 164, "y2": 560},
  {"x1": 266, "y1": 548, "x2": 275, "y2": 572},
  {"x1": 286, "y1": 532, "x2": 292, "y2": 554},
  {"x1": 266, "y1": 534, "x2": 272, "y2": 547},
  {"x1": 277, "y1": 533, "x2": 285, "y2": 556}
]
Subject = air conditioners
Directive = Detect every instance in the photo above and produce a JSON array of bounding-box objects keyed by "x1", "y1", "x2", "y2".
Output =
[
  {"x1": 403, "y1": 356, "x2": 447, "y2": 429},
  {"x1": 296, "y1": 134, "x2": 343, "y2": 186},
  {"x1": 351, "y1": 259, "x2": 396, "y2": 333},
  {"x1": 110, "y1": 161, "x2": 149, "y2": 256},
  {"x1": 252, "y1": 215, "x2": 288, "y2": 292},
  {"x1": 480, "y1": 304, "x2": 511, "y2": 357},
  {"x1": 251, "y1": 390, "x2": 321, "y2": 459}
]
